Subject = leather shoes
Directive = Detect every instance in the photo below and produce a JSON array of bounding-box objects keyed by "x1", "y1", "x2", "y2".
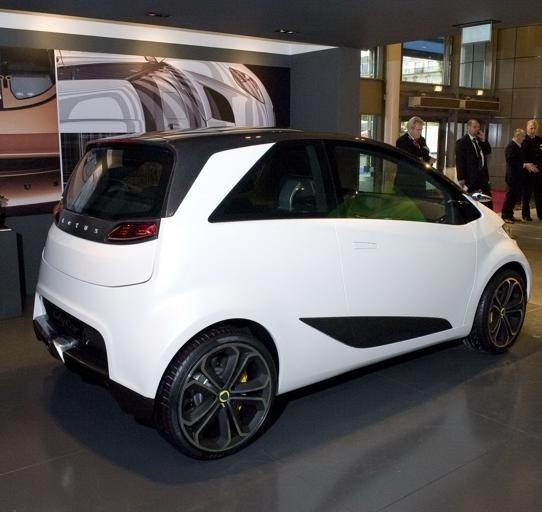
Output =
[
  {"x1": 504, "y1": 216, "x2": 516, "y2": 224},
  {"x1": 522, "y1": 215, "x2": 532, "y2": 221}
]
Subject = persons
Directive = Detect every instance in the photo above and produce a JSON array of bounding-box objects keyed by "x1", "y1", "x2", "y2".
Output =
[
  {"x1": 455, "y1": 118, "x2": 494, "y2": 214},
  {"x1": 520, "y1": 119, "x2": 542, "y2": 223},
  {"x1": 393, "y1": 116, "x2": 439, "y2": 198},
  {"x1": 501, "y1": 127, "x2": 538, "y2": 223}
]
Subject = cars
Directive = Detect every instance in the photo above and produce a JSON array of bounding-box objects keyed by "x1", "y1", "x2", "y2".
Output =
[{"x1": 33, "y1": 127, "x2": 533, "y2": 460}]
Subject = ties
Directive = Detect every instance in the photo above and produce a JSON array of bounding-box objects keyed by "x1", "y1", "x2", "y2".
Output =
[
  {"x1": 413, "y1": 140, "x2": 420, "y2": 148},
  {"x1": 473, "y1": 138, "x2": 482, "y2": 167}
]
